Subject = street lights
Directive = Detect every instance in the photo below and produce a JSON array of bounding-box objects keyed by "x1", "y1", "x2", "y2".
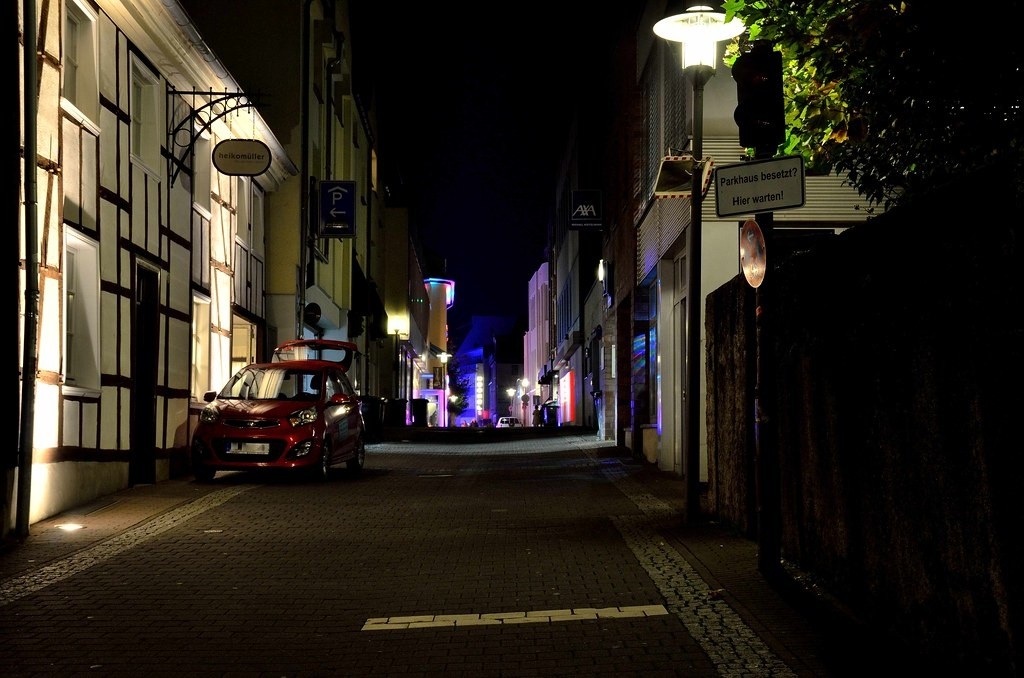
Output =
[{"x1": 652, "y1": 1, "x2": 751, "y2": 538}]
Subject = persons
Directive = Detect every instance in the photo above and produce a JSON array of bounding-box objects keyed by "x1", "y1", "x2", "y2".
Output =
[
  {"x1": 531, "y1": 405, "x2": 540, "y2": 428},
  {"x1": 460, "y1": 420, "x2": 468, "y2": 427},
  {"x1": 539, "y1": 406, "x2": 547, "y2": 428},
  {"x1": 469, "y1": 419, "x2": 479, "y2": 433}
]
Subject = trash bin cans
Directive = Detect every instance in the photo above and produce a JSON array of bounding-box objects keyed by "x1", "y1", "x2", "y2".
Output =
[{"x1": 360, "y1": 395, "x2": 430, "y2": 433}]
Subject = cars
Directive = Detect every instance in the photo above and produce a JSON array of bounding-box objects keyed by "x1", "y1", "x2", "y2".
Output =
[{"x1": 192, "y1": 335, "x2": 366, "y2": 481}]
